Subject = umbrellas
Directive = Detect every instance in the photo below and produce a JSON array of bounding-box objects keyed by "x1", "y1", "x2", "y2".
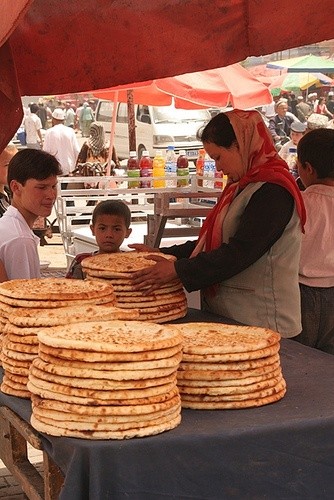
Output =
[
  {"x1": 246, "y1": 64, "x2": 334, "y2": 103},
  {"x1": 0, "y1": 0, "x2": 334, "y2": 154},
  {"x1": 92, "y1": 64, "x2": 273, "y2": 110}
]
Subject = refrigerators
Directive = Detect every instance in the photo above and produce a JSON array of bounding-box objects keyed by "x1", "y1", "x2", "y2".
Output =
[{"x1": 69, "y1": 222, "x2": 201, "y2": 309}]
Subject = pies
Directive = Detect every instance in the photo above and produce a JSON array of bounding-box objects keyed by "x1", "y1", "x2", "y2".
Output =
[{"x1": 0, "y1": 251, "x2": 286, "y2": 440}]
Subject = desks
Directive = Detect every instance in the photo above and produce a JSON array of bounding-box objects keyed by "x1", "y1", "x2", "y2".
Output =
[{"x1": 0, "y1": 306, "x2": 334, "y2": 500}]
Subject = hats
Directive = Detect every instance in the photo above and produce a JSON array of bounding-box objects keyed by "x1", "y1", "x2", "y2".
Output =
[
  {"x1": 307, "y1": 93, "x2": 317, "y2": 98},
  {"x1": 308, "y1": 113, "x2": 328, "y2": 130},
  {"x1": 291, "y1": 121, "x2": 305, "y2": 133},
  {"x1": 52, "y1": 108, "x2": 65, "y2": 120},
  {"x1": 298, "y1": 96, "x2": 303, "y2": 100}
]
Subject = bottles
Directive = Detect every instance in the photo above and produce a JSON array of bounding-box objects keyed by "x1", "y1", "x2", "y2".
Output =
[
  {"x1": 164, "y1": 145, "x2": 177, "y2": 188},
  {"x1": 195, "y1": 149, "x2": 206, "y2": 187},
  {"x1": 152, "y1": 151, "x2": 166, "y2": 188},
  {"x1": 176, "y1": 150, "x2": 190, "y2": 188},
  {"x1": 127, "y1": 150, "x2": 140, "y2": 189},
  {"x1": 139, "y1": 151, "x2": 152, "y2": 188},
  {"x1": 202, "y1": 153, "x2": 228, "y2": 191},
  {"x1": 285, "y1": 147, "x2": 297, "y2": 174}
]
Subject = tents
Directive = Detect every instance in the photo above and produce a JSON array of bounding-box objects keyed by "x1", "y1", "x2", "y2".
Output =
[{"x1": 265, "y1": 53, "x2": 334, "y2": 76}]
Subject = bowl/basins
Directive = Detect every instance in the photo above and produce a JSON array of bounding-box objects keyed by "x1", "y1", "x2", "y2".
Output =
[
  {"x1": 114, "y1": 169, "x2": 125, "y2": 177},
  {"x1": 40, "y1": 260, "x2": 51, "y2": 270}
]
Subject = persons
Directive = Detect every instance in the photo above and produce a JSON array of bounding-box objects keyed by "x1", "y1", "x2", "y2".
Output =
[
  {"x1": 65, "y1": 199, "x2": 132, "y2": 280},
  {"x1": 42, "y1": 109, "x2": 79, "y2": 190},
  {"x1": 297, "y1": 127, "x2": 334, "y2": 355},
  {"x1": 0, "y1": 142, "x2": 61, "y2": 286},
  {"x1": 128, "y1": 109, "x2": 306, "y2": 344},
  {"x1": 75, "y1": 121, "x2": 122, "y2": 206},
  {"x1": 16, "y1": 96, "x2": 96, "y2": 149},
  {"x1": 261, "y1": 86, "x2": 334, "y2": 191}
]
